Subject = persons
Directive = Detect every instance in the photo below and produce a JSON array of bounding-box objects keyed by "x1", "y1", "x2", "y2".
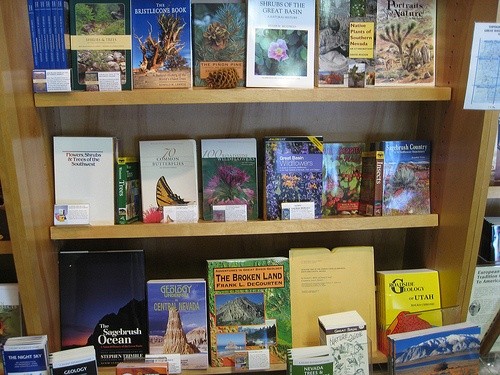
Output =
[{"x1": 319, "y1": 19, "x2": 348, "y2": 71}]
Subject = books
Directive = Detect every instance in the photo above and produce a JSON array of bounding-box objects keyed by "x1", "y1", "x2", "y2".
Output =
[
  {"x1": 48, "y1": 345, "x2": 98, "y2": 375},
  {"x1": 286, "y1": 345, "x2": 334, "y2": 375},
  {"x1": 201, "y1": 138, "x2": 258, "y2": 220},
  {"x1": 117, "y1": 157, "x2": 141, "y2": 225},
  {"x1": 387, "y1": 322, "x2": 481, "y2": 375},
  {"x1": 58, "y1": 249, "x2": 150, "y2": 367},
  {"x1": 246, "y1": 0, "x2": 315, "y2": 89},
  {"x1": 369, "y1": 139, "x2": 431, "y2": 216},
  {"x1": 358, "y1": 151, "x2": 384, "y2": 217},
  {"x1": 0, "y1": 283, "x2": 28, "y2": 337},
  {"x1": 262, "y1": 135, "x2": 324, "y2": 221},
  {"x1": 140, "y1": 139, "x2": 199, "y2": 224},
  {"x1": 316, "y1": 0, "x2": 437, "y2": 88},
  {"x1": 323, "y1": 142, "x2": 362, "y2": 217},
  {"x1": 482, "y1": 217, "x2": 500, "y2": 262},
  {"x1": 27, "y1": 0, "x2": 72, "y2": 69},
  {"x1": 147, "y1": 278, "x2": 208, "y2": 370},
  {"x1": 206, "y1": 257, "x2": 292, "y2": 367},
  {"x1": 194, "y1": 2, "x2": 246, "y2": 87},
  {"x1": 376, "y1": 269, "x2": 442, "y2": 355},
  {"x1": 54, "y1": 136, "x2": 124, "y2": 227},
  {"x1": 132, "y1": 0, "x2": 193, "y2": 89},
  {"x1": 2, "y1": 334, "x2": 49, "y2": 375},
  {"x1": 71, "y1": 0, "x2": 133, "y2": 91}
]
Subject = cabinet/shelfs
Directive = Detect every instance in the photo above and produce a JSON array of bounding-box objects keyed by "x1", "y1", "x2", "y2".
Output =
[{"x1": 0, "y1": 0, "x2": 500, "y2": 375}]
